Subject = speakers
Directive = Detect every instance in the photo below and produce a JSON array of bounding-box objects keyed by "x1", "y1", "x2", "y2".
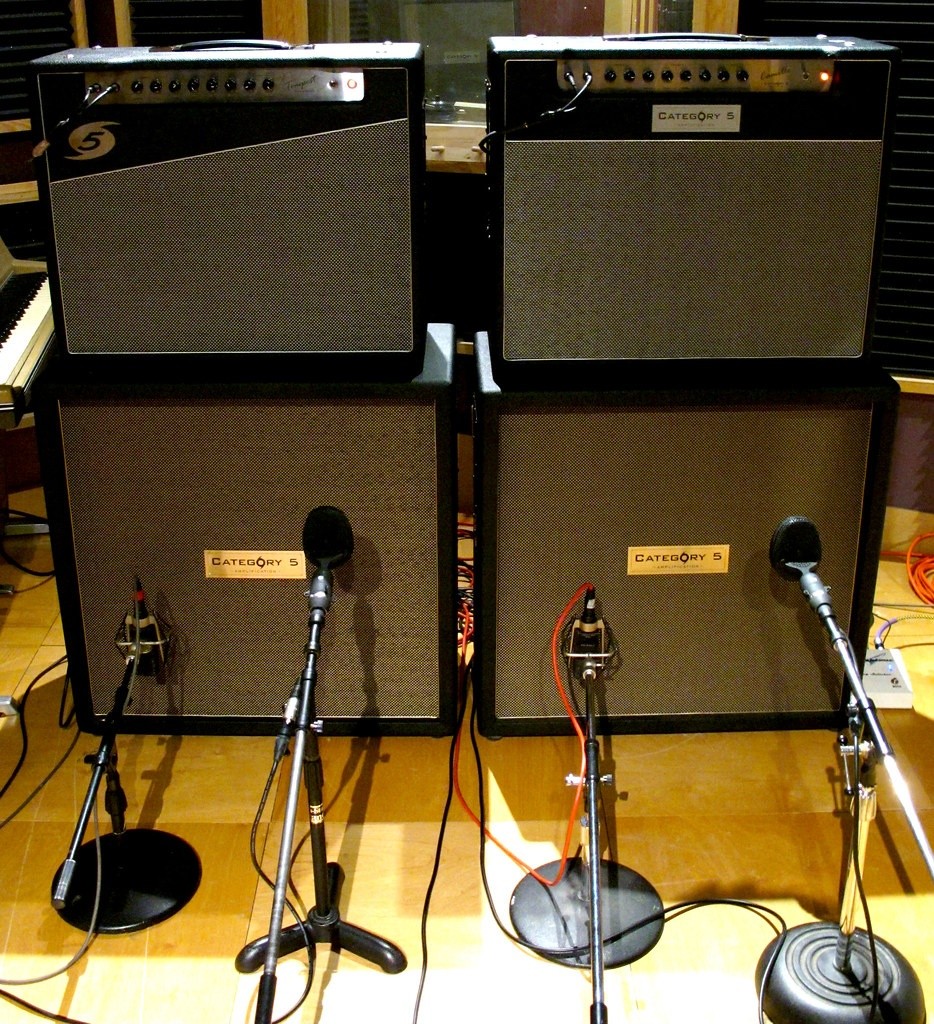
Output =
[
  {"x1": 472, "y1": 29, "x2": 905, "y2": 739},
  {"x1": 23, "y1": 38, "x2": 461, "y2": 734}
]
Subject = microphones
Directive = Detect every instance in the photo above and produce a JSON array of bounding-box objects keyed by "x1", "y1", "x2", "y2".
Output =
[
  {"x1": 768, "y1": 514, "x2": 837, "y2": 625},
  {"x1": 130, "y1": 576, "x2": 158, "y2": 677},
  {"x1": 577, "y1": 584, "x2": 601, "y2": 653},
  {"x1": 302, "y1": 506, "x2": 355, "y2": 613}
]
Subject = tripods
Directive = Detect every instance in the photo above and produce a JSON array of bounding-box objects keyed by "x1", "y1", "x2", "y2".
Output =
[{"x1": 233, "y1": 617, "x2": 409, "y2": 1023}]
